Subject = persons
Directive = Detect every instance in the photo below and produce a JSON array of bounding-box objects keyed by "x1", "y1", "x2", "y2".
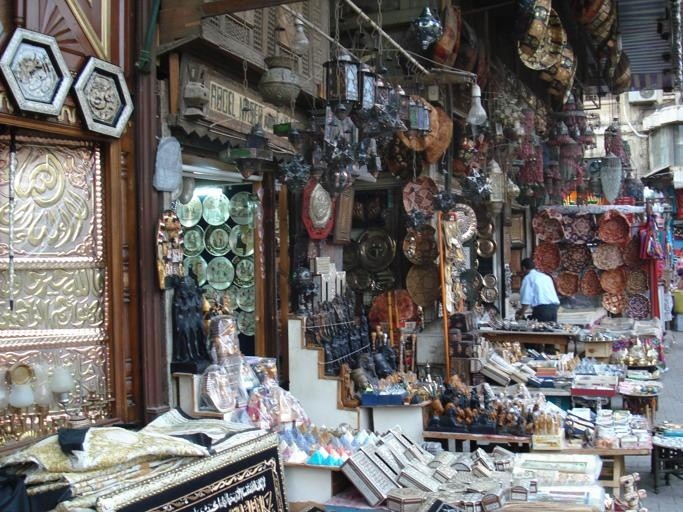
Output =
[{"x1": 516, "y1": 258, "x2": 560, "y2": 355}]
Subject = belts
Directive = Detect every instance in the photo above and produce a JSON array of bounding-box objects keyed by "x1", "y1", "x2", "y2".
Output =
[{"x1": 533, "y1": 303, "x2": 559, "y2": 310}]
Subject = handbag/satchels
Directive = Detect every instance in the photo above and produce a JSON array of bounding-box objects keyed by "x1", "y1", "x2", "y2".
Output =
[{"x1": 640, "y1": 202, "x2": 667, "y2": 260}]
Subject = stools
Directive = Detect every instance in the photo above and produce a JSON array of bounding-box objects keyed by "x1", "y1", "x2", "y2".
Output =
[{"x1": 618, "y1": 385, "x2": 662, "y2": 426}]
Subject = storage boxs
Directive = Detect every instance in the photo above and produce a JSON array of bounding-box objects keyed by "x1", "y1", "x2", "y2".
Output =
[
  {"x1": 671, "y1": 292, "x2": 683, "y2": 313},
  {"x1": 673, "y1": 313, "x2": 683, "y2": 332},
  {"x1": 584, "y1": 340, "x2": 614, "y2": 364}
]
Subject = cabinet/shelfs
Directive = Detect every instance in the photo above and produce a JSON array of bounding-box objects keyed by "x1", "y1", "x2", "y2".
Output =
[
  {"x1": 360, "y1": 399, "x2": 433, "y2": 447},
  {"x1": 173, "y1": 355, "x2": 351, "y2": 505},
  {"x1": 286, "y1": 314, "x2": 369, "y2": 431}
]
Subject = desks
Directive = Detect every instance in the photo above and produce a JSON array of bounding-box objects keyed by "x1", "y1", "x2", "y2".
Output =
[
  {"x1": 480, "y1": 332, "x2": 571, "y2": 355},
  {"x1": 422, "y1": 428, "x2": 532, "y2": 453},
  {"x1": 528, "y1": 438, "x2": 652, "y2": 500}
]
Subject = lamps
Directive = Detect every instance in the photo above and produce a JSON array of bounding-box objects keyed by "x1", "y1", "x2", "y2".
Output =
[
  {"x1": 0, "y1": 384, "x2": 9, "y2": 448},
  {"x1": 468, "y1": 83, "x2": 487, "y2": 125},
  {"x1": 33, "y1": 383, "x2": 56, "y2": 438},
  {"x1": 50, "y1": 366, "x2": 91, "y2": 430},
  {"x1": 8, "y1": 384, "x2": 34, "y2": 441},
  {"x1": 291, "y1": 14, "x2": 309, "y2": 55}
]
